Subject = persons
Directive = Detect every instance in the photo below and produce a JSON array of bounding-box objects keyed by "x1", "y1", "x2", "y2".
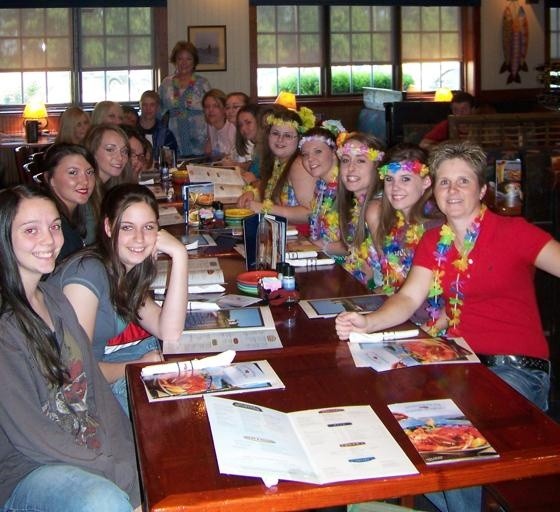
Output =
[
  {"x1": 202, "y1": 89, "x2": 236, "y2": 158},
  {"x1": 45, "y1": 183, "x2": 189, "y2": 418},
  {"x1": 120, "y1": 104, "x2": 139, "y2": 127},
  {"x1": 55, "y1": 107, "x2": 91, "y2": 147},
  {"x1": 335, "y1": 139, "x2": 560, "y2": 511},
  {"x1": 137, "y1": 91, "x2": 179, "y2": 160},
  {"x1": 298, "y1": 118, "x2": 348, "y2": 257},
  {"x1": 91, "y1": 101, "x2": 123, "y2": 125},
  {"x1": 379, "y1": 146, "x2": 449, "y2": 336},
  {"x1": 85, "y1": 123, "x2": 130, "y2": 184},
  {"x1": 237, "y1": 104, "x2": 263, "y2": 158},
  {"x1": 43, "y1": 142, "x2": 100, "y2": 258},
  {"x1": 334, "y1": 132, "x2": 389, "y2": 296},
  {"x1": 0, "y1": 184, "x2": 145, "y2": 512},
  {"x1": 238, "y1": 107, "x2": 320, "y2": 225},
  {"x1": 226, "y1": 92, "x2": 250, "y2": 126},
  {"x1": 120, "y1": 123, "x2": 146, "y2": 171},
  {"x1": 159, "y1": 41, "x2": 211, "y2": 157},
  {"x1": 420, "y1": 93, "x2": 478, "y2": 151}
]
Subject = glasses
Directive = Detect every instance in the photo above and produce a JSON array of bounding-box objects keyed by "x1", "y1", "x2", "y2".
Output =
[
  {"x1": 129, "y1": 150, "x2": 145, "y2": 162},
  {"x1": 269, "y1": 129, "x2": 301, "y2": 141}
]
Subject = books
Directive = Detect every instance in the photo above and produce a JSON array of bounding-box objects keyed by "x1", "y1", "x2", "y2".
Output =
[
  {"x1": 386, "y1": 397, "x2": 501, "y2": 466},
  {"x1": 201, "y1": 393, "x2": 420, "y2": 486},
  {"x1": 347, "y1": 335, "x2": 482, "y2": 373},
  {"x1": 185, "y1": 165, "x2": 246, "y2": 204},
  {"x1": 147, "y1": 185, "x2": 166, "y2": 200},
  {"x1": 233, "y1": 214, "x2": 333, "y2": 272},
  {"x1": 161, "y1": 304, "x2": 284, "y2": 355},
  {"x1": 148, "y1": 257, "x2": 227, "y2": 294},
  {"x1": 297, "y1": 293, "x2": 388, "y2": 321},
  {"x1": 495, "y1": 158, "x2": 522, "y2": 199},
  {"x1": 158, "y1": 206, "x2": 185, "y2": 227},
  {"x1": 140, "y1": 359, "x2": 286, "y2": 404}
]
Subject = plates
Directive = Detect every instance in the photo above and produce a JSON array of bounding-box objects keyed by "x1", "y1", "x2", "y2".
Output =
[
  {"x1": 224, "y1": 209, "x2": 255, "y2": 225},
  {"x1": 237, "y1": 270, "x2": 278, "y2": 295}
]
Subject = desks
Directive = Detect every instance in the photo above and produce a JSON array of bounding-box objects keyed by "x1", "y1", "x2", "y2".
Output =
[{"x1": 126, "y1": 252, "x2": 559, "y2": 512}]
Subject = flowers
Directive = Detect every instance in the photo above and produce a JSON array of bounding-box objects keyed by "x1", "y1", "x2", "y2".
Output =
[
  {"x1": 336, "y1": 132, "x2": 385, "y2": 162},
  {"x1": 260, "y1": 199, "x2": 274, "y2": 215},
  {"x1": 377, "y1": 162, "x2": 429, "y2": 180},
  {"x1": 265, "y1": 159, "x2": 288, "y2": 205},
  {"x1": 379, "y1": 210, "x2": 424, "y2": 297},
  {"x1": 297, "y1": 134, "x2": 337, "y2": 152},
  {"x1": 241, "y1": 184, "x2": 259, "y2": 201},
  {"x1": 425, "y1": 203, "x2": 486, "y2": 340},
  {"x1": 309, "y1": 166, "x2": 339, "y2": 241},
  {"x1": 267, "y1": 106, "x2": 317, "y2": 133},
  {"x1": 342, "y1": 193, "x2": 375, "y2": 292}
]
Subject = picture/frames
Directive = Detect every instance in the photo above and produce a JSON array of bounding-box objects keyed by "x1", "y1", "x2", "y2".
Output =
[{"x1": 187, "y1": 25, "x2": 228, "y2": 73}]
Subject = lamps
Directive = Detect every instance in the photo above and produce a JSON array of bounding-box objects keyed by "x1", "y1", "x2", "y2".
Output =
[
  {"x1": 433, "y1": 87, "x2": 454, "y2": 103},
  {"x1": 21, "y1": 98, "x2": 48, "y2": 121},
  {"x1": 275, "y1": 91, "x2": 298, "y2": 111}
]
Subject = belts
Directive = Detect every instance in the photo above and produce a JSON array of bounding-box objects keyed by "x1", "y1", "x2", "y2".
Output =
[{"x1": 477, "y1": 353, "x2": 552, "y2": 372}]
